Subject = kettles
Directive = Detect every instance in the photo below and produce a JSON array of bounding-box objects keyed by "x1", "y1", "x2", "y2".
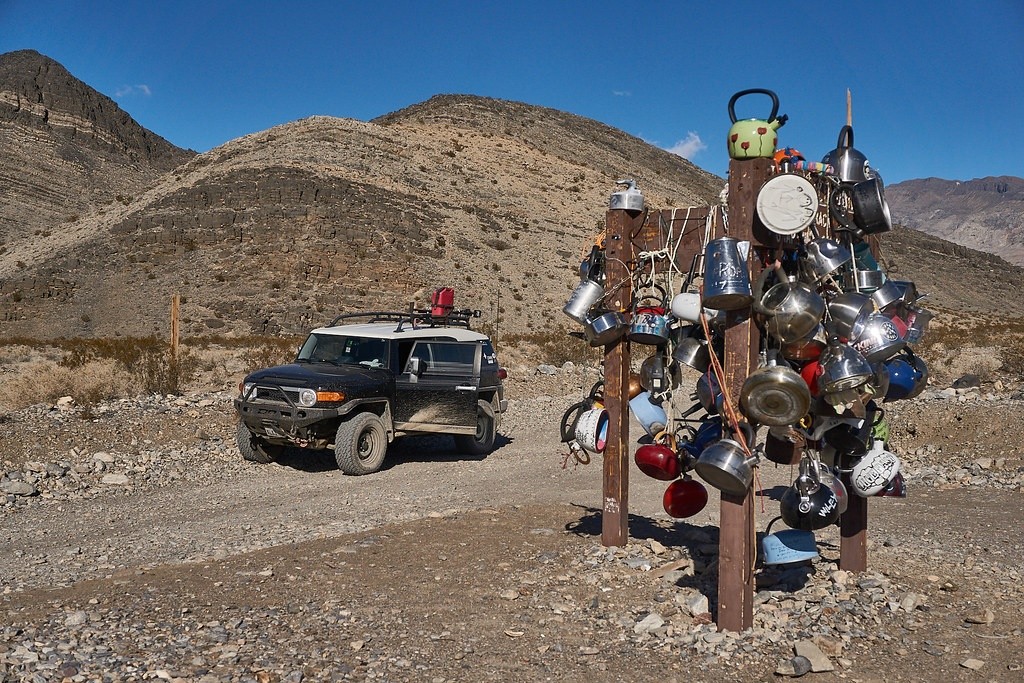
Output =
[{"x1": 557, "y1": 88, "x2": 928, "y2": 567}]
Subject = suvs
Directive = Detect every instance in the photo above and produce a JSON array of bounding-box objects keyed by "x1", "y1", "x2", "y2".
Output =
[{"x1": 234, "y1": 302, "x2": 508, "y2": 476}]
